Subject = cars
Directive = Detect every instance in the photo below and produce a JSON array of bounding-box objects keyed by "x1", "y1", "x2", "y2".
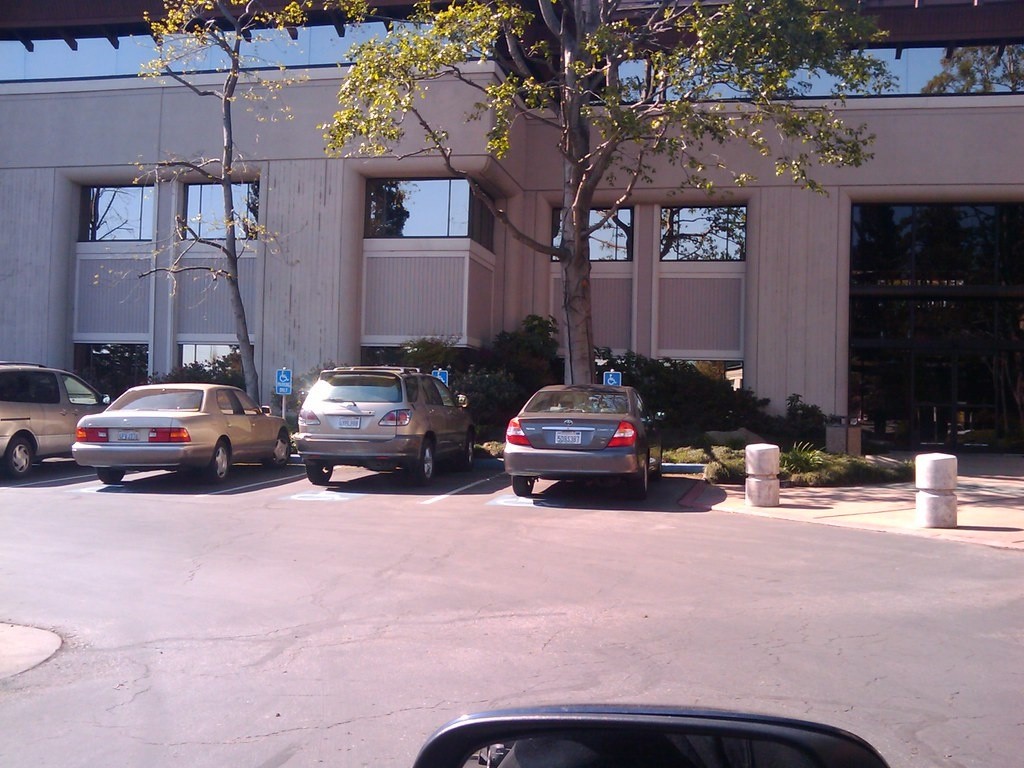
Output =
[
  {"x1": 477, "y1": 740, "x2": 518, "y2": 767},
  {"x1": 72, "y1": 383, "x2": 292, "y2": 486},
  {"x1": 501, "y1": 383, "x2": 668, "y2": 502}
]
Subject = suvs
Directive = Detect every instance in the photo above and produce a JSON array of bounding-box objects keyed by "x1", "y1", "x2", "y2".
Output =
[
  {"x1": 291, "y1": 365, "x2": 476, "y2": 486},
  {"x1": 0, "y1": 360, "x2": 116, "y2": 479}
]
